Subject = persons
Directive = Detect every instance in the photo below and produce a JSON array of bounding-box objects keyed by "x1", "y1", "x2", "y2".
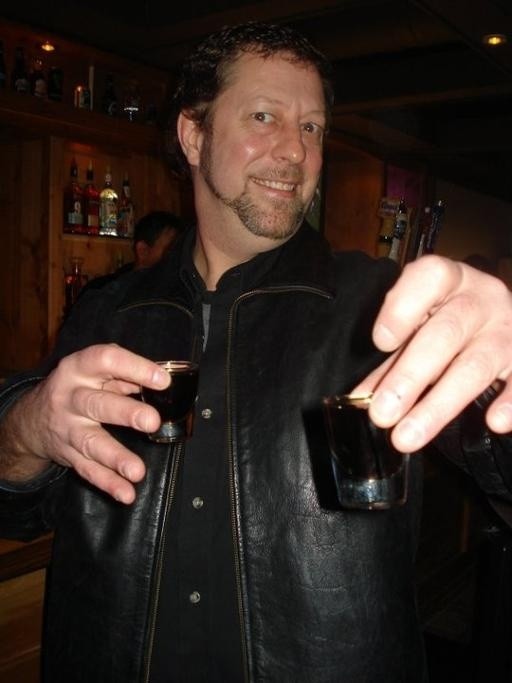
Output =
[
  {"x1": 1, "y1": 17, "x2": 510, "y2": 680},
  {"x1": 107, "y1": 206, "x2": 186, "y2": 277}
]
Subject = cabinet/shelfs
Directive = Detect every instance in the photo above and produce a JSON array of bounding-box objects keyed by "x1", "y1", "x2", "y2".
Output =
[{"x1": 1, "y1": 21, "x2": 176, "y2": 369}]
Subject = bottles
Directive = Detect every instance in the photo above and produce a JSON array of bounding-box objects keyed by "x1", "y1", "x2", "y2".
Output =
[
  {"x1": 11, "y1": 42, "x2": 30, "y2": 91},
  {"x1": 59, "y1": 251, "x2": 91, "y2": 320},
  {"x1": 86, "y1": 159, "x2": 100, "y2": 234},
  {"x1": 119, "y1": 168, "x2": 136, "y2": 238},
  {"x1": 100, "y1": 162, "x2": 120, "y2": 235},
  {"x1": 100, "y1": 68, "x2": 120, "y2": 116},
  {"x1": 66, "y1": 156, "x2": 86, "y2": 233},
  {"x1": 31, "y1": 57, "x2": 48, "y2": 96},
  {"x1": 0, "y1": 37, "x2": 8, "y2": 86}
]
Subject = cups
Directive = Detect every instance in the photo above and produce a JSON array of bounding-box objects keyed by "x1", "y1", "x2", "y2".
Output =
[
  {"x1": 139, "y1": 360, "x2": 199, "y2": 443},
  {"x1": 322, "y1": 395, "x2": 410, "y2": 510}
]
